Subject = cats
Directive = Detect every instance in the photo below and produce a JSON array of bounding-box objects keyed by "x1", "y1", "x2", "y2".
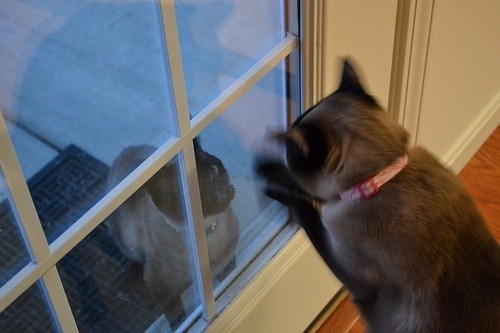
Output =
[
  {"x1": 80, "y1": 113, "x2": 239, "y2": 333},
  {"x1": 253, "y1": 57, "x2": 500, "y2": 333}
]
